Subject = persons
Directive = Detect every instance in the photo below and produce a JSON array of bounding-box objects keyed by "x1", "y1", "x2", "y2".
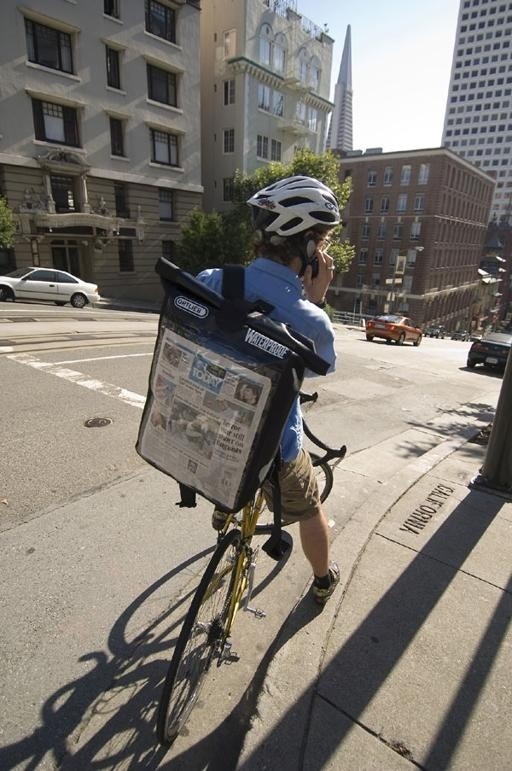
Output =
[{"x1": 191, "y1": 175, "x2": 342, "y2": 604}]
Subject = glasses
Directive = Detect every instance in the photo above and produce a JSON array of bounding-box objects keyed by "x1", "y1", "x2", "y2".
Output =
[{"x1": 304, "y1": 231, "x2": 331, "y2": 252}]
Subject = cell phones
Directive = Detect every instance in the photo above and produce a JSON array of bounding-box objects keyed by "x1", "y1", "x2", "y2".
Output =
[{"x1": 302, "y1": 234, "x2": 318, "y2": 279}]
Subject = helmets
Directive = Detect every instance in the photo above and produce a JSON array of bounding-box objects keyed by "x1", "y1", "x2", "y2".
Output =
[{"x1": 246, "y1": 176, "x2": 341, "y2": 247}]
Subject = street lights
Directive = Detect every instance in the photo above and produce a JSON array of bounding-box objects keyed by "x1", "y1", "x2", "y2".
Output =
[{"x1": 387, "y1": 246, "x2": 424, "y2": 315}]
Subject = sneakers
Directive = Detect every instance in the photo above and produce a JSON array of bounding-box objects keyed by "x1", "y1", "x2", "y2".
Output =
[
  {"x1": 311, "y1": 560, "x2": 340, "y2": 603},
  {"x1": 212, "y1": 511, "x2": 228, "y2": 530}
]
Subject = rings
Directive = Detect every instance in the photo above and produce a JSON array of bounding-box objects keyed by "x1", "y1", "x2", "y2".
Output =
[{"x1": 327, "y1": 265, "x2": 337, "y2": 270}]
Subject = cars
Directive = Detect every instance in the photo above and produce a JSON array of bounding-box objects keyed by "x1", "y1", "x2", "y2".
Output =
[
  {"x1": 426, "y1": 324, "x2": 446, "y2": 339},
  {"x1": 365, "y1": 314, "x2": 422, "y2": 346},
  {"x1": 0, "y1": 266, "x2": 101, "y2": 309},
  {"x1": 451, "y1": 330, "x2": 469, "y2": 341},
  {"x1": 466, "y1": 332, "x2": 511, "y2": 373}
]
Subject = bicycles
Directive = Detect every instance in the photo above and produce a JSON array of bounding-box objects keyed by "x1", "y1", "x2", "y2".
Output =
[{"x1": 155, "y1": 390, "x2": 347, "y2": 747}]
